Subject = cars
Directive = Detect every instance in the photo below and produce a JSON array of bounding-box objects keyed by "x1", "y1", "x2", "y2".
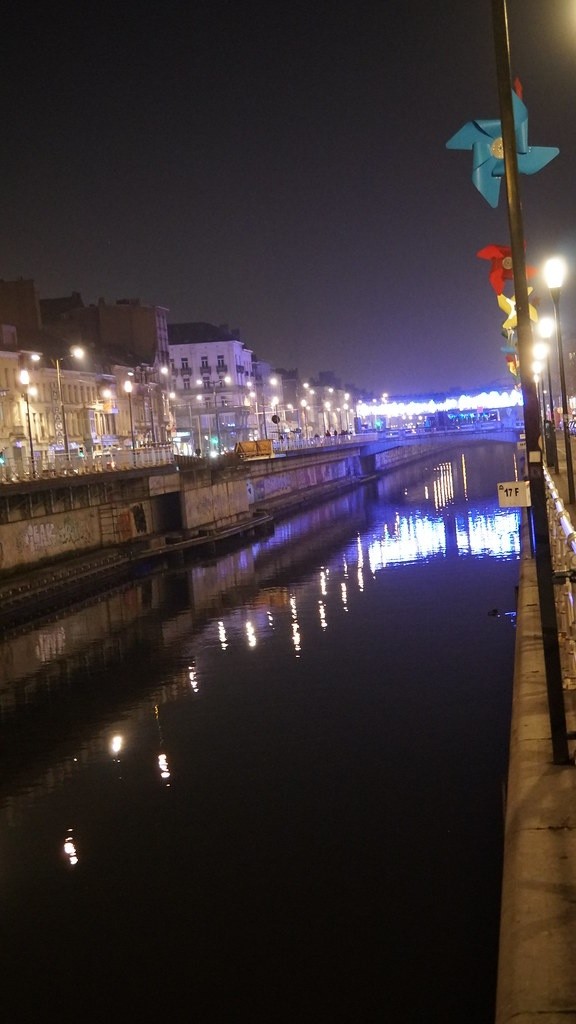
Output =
[{"x1": 559, "y1": 418, "x2": 576, "y2": 437}]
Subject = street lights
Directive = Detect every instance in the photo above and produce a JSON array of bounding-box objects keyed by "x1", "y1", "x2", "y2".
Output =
[
  {"x1": 31, "y1": 346, "x2": 85, "y2": 460},
  {"x1": 246, "y1": 377, "x2": 358, "y2": 442},
  {"x1": 124, "y1": 380, "x2": 136, "y2": 451},
  {"x1": 537, "y1": 317, "x2": 561, "y2": 476},
  {"x1": 197, "y1": 376, "x2": 232, "y2": 456},
  {"x1": 541, "y1": 253, "x2": 575, "y2": 509},
  {"x1": 20, "y1": 369, "x2": 37, "y2": 480},
  {"x1": 530, "y1": 343, "x2": 551, "y2": 467},
  {"x1": 170, "y1": 391, "x2": 201, "y2": 440},
  {"x1": 128, "y1": 365, "x2": 168, "y2": 447}
]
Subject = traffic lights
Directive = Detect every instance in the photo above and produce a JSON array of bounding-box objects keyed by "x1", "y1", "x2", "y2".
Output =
[
  {"x1": 0, "y1": 451, "x2": 6, "y2": 466},
  {"x1": 78, "y1": 446, "x2": 84, "y2": 458}
]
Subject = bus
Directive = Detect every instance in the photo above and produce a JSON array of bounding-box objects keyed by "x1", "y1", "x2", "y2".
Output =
[{"x1": 423, "y1": 408, "x2": 502, "y2": 434}]
